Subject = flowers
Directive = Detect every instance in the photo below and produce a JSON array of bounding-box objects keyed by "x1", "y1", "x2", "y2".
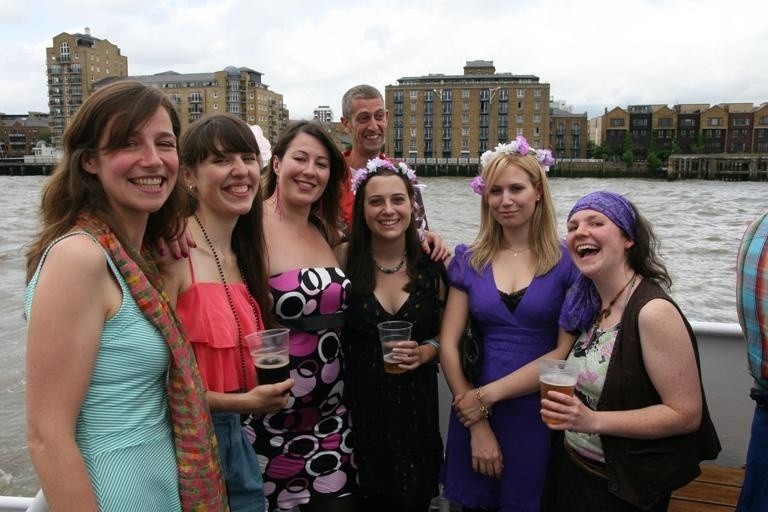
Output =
[
  {"x1": 351, "y1": 155, "x2": 426, "y2": 197},
  {"x1": 471, "y1": 135, "x2": 553, "y2": 195}
]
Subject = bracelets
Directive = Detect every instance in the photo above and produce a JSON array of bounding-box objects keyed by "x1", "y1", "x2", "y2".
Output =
[{"x1": 476, "y1": 386, "x2": 491, "y2": 417}]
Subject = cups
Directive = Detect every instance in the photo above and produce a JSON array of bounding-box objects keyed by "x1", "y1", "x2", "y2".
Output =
[
  {"x1": 376, "y1": 319, "x2": 412, "y2": 375},
  {"x1": 536, "y1": 357, "x2": 582, "y2": 425},
  {"x1": 244, "y1": 326, "x2": 291, "y2": 385}
]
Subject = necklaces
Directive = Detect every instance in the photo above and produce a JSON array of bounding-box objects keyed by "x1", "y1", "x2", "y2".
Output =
[
  {"x1": 505, "y1": 244, "x2": 529, "y2": 257},
  {"x1": 193, "y1": 209, "x2": 260, "y2": 392},
  {"x1": 370, "y1": 243, "x2": 407, "y2": 273},
  {"x1": 597, "y1": 272, "x2": 635, "y2": 322}
]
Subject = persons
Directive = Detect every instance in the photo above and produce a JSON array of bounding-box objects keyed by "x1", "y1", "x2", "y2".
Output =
[
  {"x1": 151, "y1": 113, "x2": 294, "y2": 511},
  {"x1": 160, "y1": 120, "x2": 449, "y2": 511},
  {"x1": 735, "y1": 214, "x2": 767, "y2": 511},
  {"x1": 341, "y1": 160, "x2": 452, "y2": 511},
  {"x1": 438, "y1": 139, "x2": 585, "y2": 512},
  {"x1": 539, "y1": 192, "x2": 721, "y2": 510},
  {"x1": 333, "y1": 86, "x2": 429, "y2": 237},
  {"x1": 24, "y1": 81, "x2": 226, "y2": 512}
]
